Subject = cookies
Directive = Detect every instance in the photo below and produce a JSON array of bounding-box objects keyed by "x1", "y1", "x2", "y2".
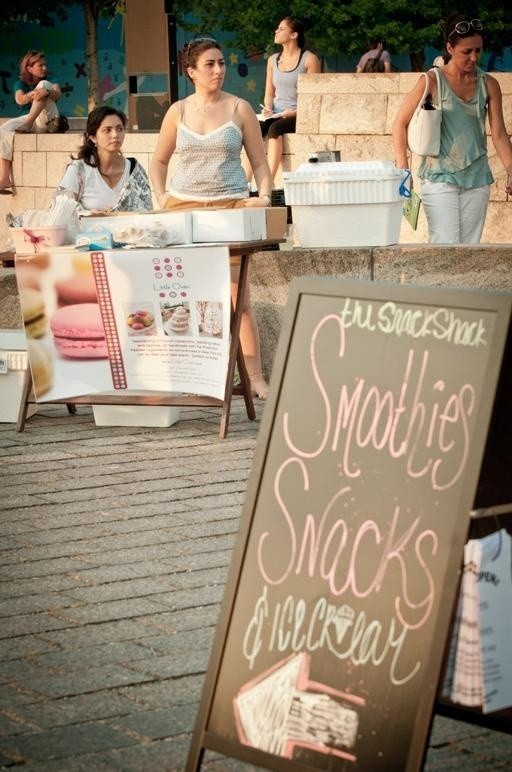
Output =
[{"x1": 21, "y1": 273, "x2": 154, "y2": 398}]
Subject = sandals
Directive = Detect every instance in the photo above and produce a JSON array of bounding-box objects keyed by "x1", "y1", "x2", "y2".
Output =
[{"x1": 248, "y1": 373, "x2": 270, "y2": 401}]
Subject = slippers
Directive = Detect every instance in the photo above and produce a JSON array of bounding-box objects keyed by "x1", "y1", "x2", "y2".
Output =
[
  {"x1": 14, "y1": 126, "x2": 37, "y2": 134},
  {"x1": 0, "y1": 187, "x2": 12, "y2": 195}
]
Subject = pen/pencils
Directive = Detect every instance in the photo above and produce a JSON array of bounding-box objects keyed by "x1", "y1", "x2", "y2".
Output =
[
  {"x1": 259, "y1": 104, "x2": 268, "y2": 110},
  {"x1": 109, "y1": 204, "x2": 119, "y2": 212}
]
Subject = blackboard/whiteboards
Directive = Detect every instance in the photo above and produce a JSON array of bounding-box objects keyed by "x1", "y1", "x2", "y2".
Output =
[{"x1": 200, "y1": 276, "x2": 512, "y2": 772}]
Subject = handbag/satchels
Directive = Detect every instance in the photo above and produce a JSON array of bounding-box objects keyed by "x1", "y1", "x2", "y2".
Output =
[{"x1": 45, "y1": 115, "x2": 69, "y2": 133}]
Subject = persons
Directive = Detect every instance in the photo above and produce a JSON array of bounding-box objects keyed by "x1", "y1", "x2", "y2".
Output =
[
  {"x1": 150, "y1": 37, "x2": 275, "y2": 402},
  {"x1": 239, "y1": 16, "x2": 321, "y2": 192},
  {"x1": 390, "y1": 9, "x2": 511, "y2": 244},
  {"x1": 1, "y1": 47, "x2": 61, "y2": 195},
  {"x1": 59, "y1": 106, "x2": 153, "y2": 219},
  {"x1": 356, "y1": 35, "x2": 391, "y2": 73}
]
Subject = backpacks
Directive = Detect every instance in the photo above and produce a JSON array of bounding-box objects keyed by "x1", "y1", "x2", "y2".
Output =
[
  {"x1": 363, "y1": 49, "x2": 385, "y2": 73},
  {"x1": 407, "y1": 67, "x2": 442, "y2": 156}
]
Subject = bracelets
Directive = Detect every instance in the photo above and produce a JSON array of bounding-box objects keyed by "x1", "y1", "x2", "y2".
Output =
[
  {"x1": 260, "y1": 193, "x2": 274, "y2": 200},
  {"x1": 47, "y1": 88, "x2": 55, "y2": 96}
]
